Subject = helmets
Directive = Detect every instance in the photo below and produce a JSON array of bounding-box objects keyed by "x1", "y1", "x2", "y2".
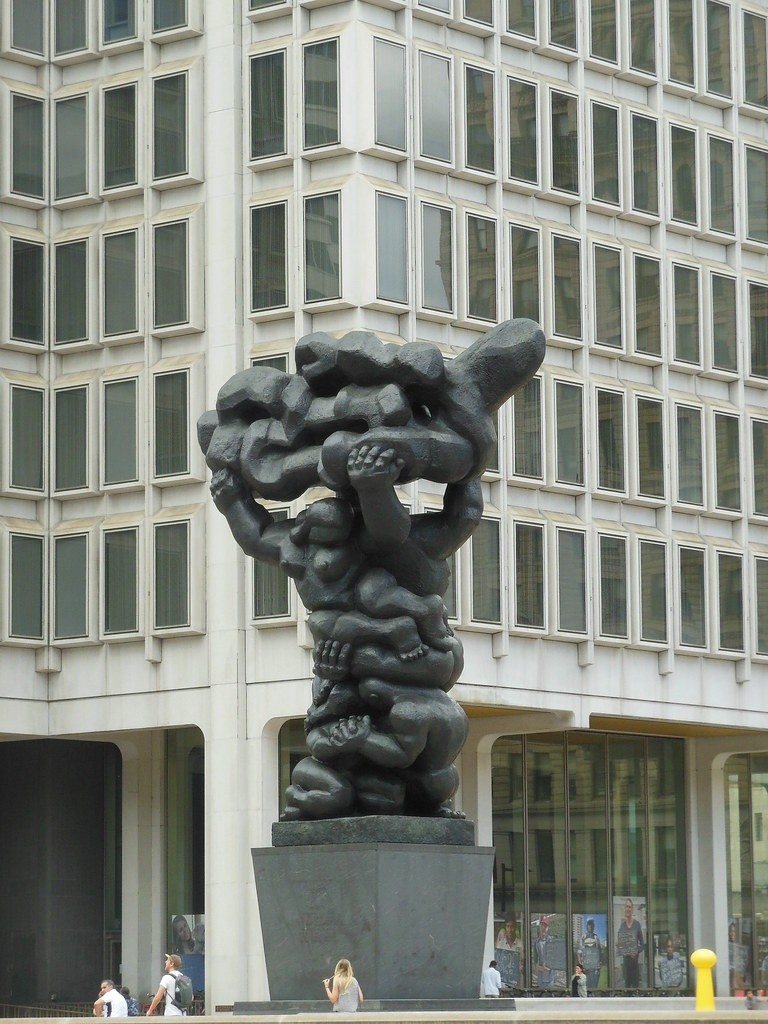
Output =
[{"x1": 540, "y1": 915, "x2": 549, "y2": 925}]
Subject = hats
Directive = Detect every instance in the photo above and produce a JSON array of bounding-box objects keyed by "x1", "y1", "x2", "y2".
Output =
[
  {"x1": 587, "y1": 918, "x2": 594, "y2": 930},
  {"x1": 164, "y1": 953, "x2": 182, "y2": 967}
]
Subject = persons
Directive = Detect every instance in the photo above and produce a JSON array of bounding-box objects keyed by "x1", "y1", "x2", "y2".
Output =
[
  {"x1": 497, "y1": 913, "x2": 524, "y2": 973},
  {"x1": 762, "y1": 956, "x2": 768, "y2": 985},
  {"x1": 534, "y1": 914, "x2": 557, "y2": 986},
  {"x1": 571, "y1": 964, "x2": 587, "y2": 998},
  {"x1": 729, "y1": 922, "x2": 738, "y2": 943},
  {"x1": 322, "y1": 959, "x2": 363, "y2": 1012},
  {"x1": 93, "y1": 980, "x2": 140, "y2": 1017},
  {"x1": 211, "y1": 445, "x2": 483, "y2": 823},
  {"x1": 482, "y1": 961, "x2": 503, "y2": 998},
  {"x1": 146, "y1": 954, "x2": 194, "y2": 1016},
  {"x1": 659, "y1": 935, "x2": 683, "y2": 988},
  {"x1": 172, "y1": 916, "x2": 195, "y2": 953},
  {"x1": 577, "y1": 917, "x2": 602, "y2": 988},
  {"x1": 616, "y1": 898, "x2": 644, "y2": 988},
  {"x1": 745, "y1": 989, "x2": 761, "y2": 1010}
]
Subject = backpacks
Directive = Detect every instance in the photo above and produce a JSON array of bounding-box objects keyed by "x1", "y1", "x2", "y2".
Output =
[{"x1": 164, "y1": 974, "x2": 193, "y2": 1008}]
